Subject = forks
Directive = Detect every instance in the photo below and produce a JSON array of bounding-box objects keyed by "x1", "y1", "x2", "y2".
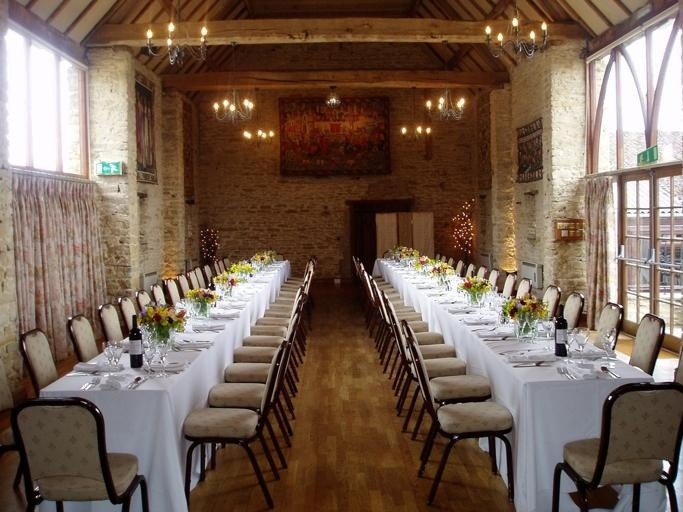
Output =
[
  {"x1": 556, "y1": 366, "x2": 572, "y2": 382},
  {"x1": 80, "y1": 377, "x2": 101, "y2": 391}
]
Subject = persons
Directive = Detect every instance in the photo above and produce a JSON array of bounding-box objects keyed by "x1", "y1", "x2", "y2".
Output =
[
  {"x1": 289, "y1": 122, "x2": 387, "y2": 174},
  {"x1": 136, "y1": 90, "x2": 154, "y2": 171}
]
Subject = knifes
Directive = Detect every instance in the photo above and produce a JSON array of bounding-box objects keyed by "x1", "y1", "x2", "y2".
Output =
[
  {"x1": 512, "y1": 364, "x2": 551, "y2": 369},
  {"x1": 132, "y1": 379, "x2": 147, "y2": 390}
]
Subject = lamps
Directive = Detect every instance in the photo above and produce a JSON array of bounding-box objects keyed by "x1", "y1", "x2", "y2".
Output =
[
  {"x1": 399, "y1": 39, "x2": 466, "y2": 144},
  {"x1": 142, "y1": 1, "x2": 212, "y2": 66},
  {"x1": 482, "y1": 0, "x2": 552, "y2": 62},
  {"x1": 210, "y1": 87, "x2": 258, "y2": 124}
]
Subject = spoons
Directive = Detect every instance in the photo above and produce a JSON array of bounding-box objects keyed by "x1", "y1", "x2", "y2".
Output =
[
  {"x1": 171, "y1": 347, "x2": 201, "y2": 352},
  {"x1": 600, "y1": 367, "x2": 620, "y2": 378},
  {"x1": 182, "y1": 339, "x2": 209, "y2": 342},
  {"x1": 483, "y1": 335, "x2": 508, "y2": 341},
  {"x1": 514, "y1": 360, "x2": 544, "y2": 367},
  {"x1": 128, "y1": 376, "x2": 141, "y2": 389}
]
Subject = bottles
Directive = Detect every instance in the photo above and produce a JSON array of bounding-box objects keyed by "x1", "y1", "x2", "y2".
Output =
[
  {"x1": 128, "y1": 314, "x2": 143, "y2": 369},
  {"x1": 553, "y1": 304, "x2": 568, "y2": 358}
]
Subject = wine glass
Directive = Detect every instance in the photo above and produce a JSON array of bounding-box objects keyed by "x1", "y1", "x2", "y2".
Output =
[
  {"x1": 99, "y1": 339, "x2": 170, "y2": 379},
  {"x1": 561, "y1": 326, "x2": 615, "y2": 369},
  {"x1": 179, "y1": 284, "x2": 226, "y2": 323},
  {"x1": 475, "y1": 292, "x2": 553, "y2": 351}
]
